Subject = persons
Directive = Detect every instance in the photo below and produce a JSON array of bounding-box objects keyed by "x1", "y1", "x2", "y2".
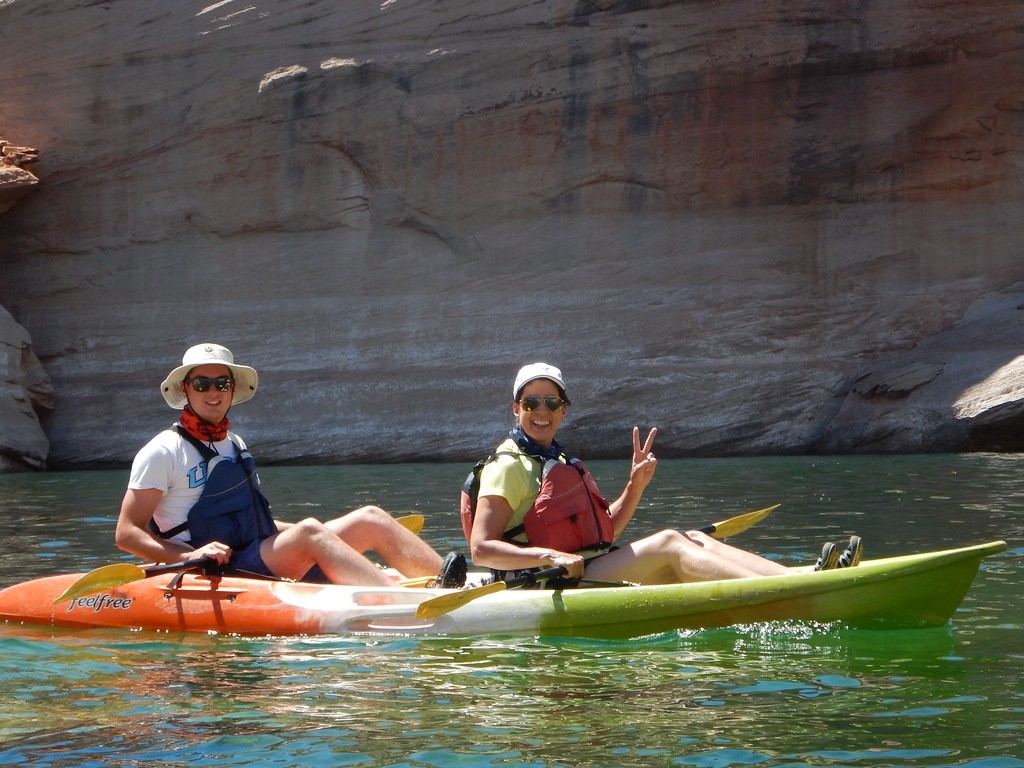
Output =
[
  {"x1": 470, "y1": 362, "x2": 864, "y2": 593},
  {"x1": 112, "y1": 343, "x2": 467, "y2": 590}
]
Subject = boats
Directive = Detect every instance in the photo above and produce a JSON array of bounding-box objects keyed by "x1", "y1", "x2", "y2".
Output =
[{"x1": 0, "y1": 536, "x2": 1011, "y2": 640}]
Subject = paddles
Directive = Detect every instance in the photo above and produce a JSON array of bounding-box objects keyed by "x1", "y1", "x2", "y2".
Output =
[
  {"x1": 418, "y1": 505, "x2": 787, "y2": 622},
  {"x1": 51, "y1": 510, "x2": 432, "y2": 605}
]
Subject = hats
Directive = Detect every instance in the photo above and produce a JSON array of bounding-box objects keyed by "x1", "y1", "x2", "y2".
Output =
[
  {"x1": 513, "y1": 362, "x2": 566, "y2": 401},
  {"x1": 161, "y1": 343, "x2": 258, "y2": 410}
]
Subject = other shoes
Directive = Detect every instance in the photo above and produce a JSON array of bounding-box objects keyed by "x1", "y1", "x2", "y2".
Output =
[
  {"x1": 838, "y1": 535, "x2": 863, "y2": 568},
  {"x1": 813, "y1": 542, "x2": 837, "y2": 571},
  {"x1": 424, "y1": 550, "x2": 467, "y2": 589}
]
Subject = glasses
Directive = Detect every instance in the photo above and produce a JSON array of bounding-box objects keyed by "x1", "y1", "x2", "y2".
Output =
[
  {"x1": 519, "y1": 395, "x2": 565, "y2": 414},
  {"x1": 187, "y1": 376, "x2": 234, "y2": 392}
]
